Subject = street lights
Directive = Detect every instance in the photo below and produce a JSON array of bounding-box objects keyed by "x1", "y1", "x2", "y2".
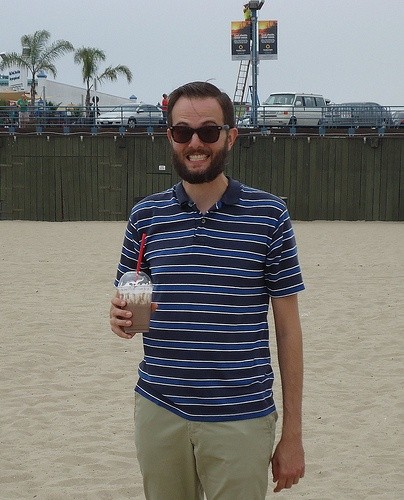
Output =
[{"x1": 247, "y1": 0, "x2": 265, "y2": 128}]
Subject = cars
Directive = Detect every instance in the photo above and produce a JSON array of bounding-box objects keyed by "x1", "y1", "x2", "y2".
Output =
[
  {"x1": 332, "y1": 102, "x2": 404, "y2": 128},
  {"x1": 96, "y1": 103, "x2": 164, "y2": 129}
]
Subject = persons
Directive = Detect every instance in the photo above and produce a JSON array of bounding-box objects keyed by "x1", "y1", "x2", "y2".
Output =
[
  {"x1": 17, "y1": 94, "x2": 29, "y2": 128},
  {"x1": 161, "y1": 94, "x2": 168, "y2": 123},
  {"x1": 110, "y1": 82, "x2": 305, "y2": 500}
]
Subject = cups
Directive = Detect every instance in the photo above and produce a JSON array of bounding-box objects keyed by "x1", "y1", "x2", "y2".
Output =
[{"x1": 116, "y1": 272, "x2": 155, "y2": 333}]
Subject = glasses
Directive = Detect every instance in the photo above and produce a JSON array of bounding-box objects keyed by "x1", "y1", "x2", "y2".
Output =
[{"x1": 168, "y1": 125, "x2": 230, "y2": 143}]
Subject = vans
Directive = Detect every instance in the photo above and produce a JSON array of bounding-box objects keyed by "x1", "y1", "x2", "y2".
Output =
[{"x1": 256, "y1": 91, "x2": 328, "y2": 128}]
